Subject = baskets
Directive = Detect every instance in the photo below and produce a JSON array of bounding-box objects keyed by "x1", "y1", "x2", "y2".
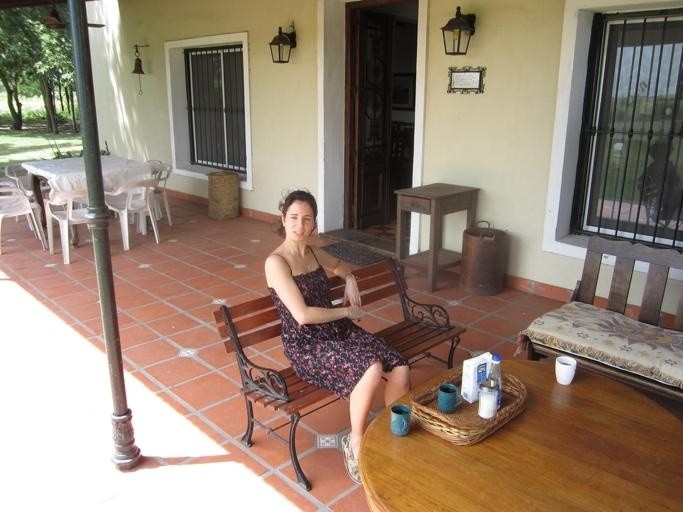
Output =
[{"x1": 408, "y1": 370, "x2": 528, "y2": 448}]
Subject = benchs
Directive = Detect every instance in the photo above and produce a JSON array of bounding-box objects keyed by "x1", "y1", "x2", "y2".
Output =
[{"x1": 212, "y1": 254, "x2": 467, "y2": 493}]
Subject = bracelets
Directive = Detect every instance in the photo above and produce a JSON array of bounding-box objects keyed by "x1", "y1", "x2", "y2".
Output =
[{"x1": 345, "y1": 273, "x2": 354, "y2": 280}]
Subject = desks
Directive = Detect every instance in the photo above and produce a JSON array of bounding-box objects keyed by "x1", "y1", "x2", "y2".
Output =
[
  {"x1": 21, "y1": 156, "x2": 151, "y2": 249},
  {"x1": 392, "y1": 182, "x2": 480, "y2": 294}
]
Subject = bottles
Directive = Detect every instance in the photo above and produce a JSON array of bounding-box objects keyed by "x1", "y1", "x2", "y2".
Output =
[{"x1": 487, "y1": 355, "x2": 500, "y2": 409}]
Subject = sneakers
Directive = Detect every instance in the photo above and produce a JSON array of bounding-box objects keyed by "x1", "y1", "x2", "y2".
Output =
[{"x1": 340, "y1": 434, "x2": 363, "y2": 485}]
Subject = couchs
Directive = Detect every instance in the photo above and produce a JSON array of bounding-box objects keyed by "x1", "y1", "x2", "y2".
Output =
[{"x1": 513, "y1": 235, "x2": 683, "y2": 401}]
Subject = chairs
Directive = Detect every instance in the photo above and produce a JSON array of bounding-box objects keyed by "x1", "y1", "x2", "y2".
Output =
[
  {"x1": 42, "y1": 189, "x2": 91, "y2": 265},
  {"x1": 1, "y1": 195, "x2": 48, "y2": 256},
  {"x1": 4, "y1": 162, "x2": 53, "y2": 224},
  {"x1": 144, "y1": 159, "x2": 163, "y2": 221},
  {"x1": 104, "y1": 179, "x2": 160, "y2": 251},
  {"x1": 151, "y1": 163, "x2": 174, "y2": 227},
  {"x1": 0, "y1": 181, "x2": 34, "y2": 231}
]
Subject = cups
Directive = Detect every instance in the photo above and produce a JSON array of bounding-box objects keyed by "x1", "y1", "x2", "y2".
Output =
[
  {"x1": 437, "y1": 383, "x2": 464, "y2": 414},
  {"x1": 478, "y1": 379, "x2": 499, "y2": 419},
  {"x1": 390, "y1": 403, "x2": 412, "y2": 437},
  {"x1": 554, "y1": 355, "x2": 578, "y2": 386}
]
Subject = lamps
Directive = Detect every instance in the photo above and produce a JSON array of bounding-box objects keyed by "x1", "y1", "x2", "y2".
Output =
[
  {"x1": 269, "y1": 21, "x2": 297, "y2": 63},
  {"x1": 441, "y1": 6, "x2": 476, "y2": 56}
]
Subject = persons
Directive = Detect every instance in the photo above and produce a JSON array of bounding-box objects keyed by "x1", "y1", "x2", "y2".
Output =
[{"x1": 264, "y1": 185, "x2": 412, "y2": 485}]
